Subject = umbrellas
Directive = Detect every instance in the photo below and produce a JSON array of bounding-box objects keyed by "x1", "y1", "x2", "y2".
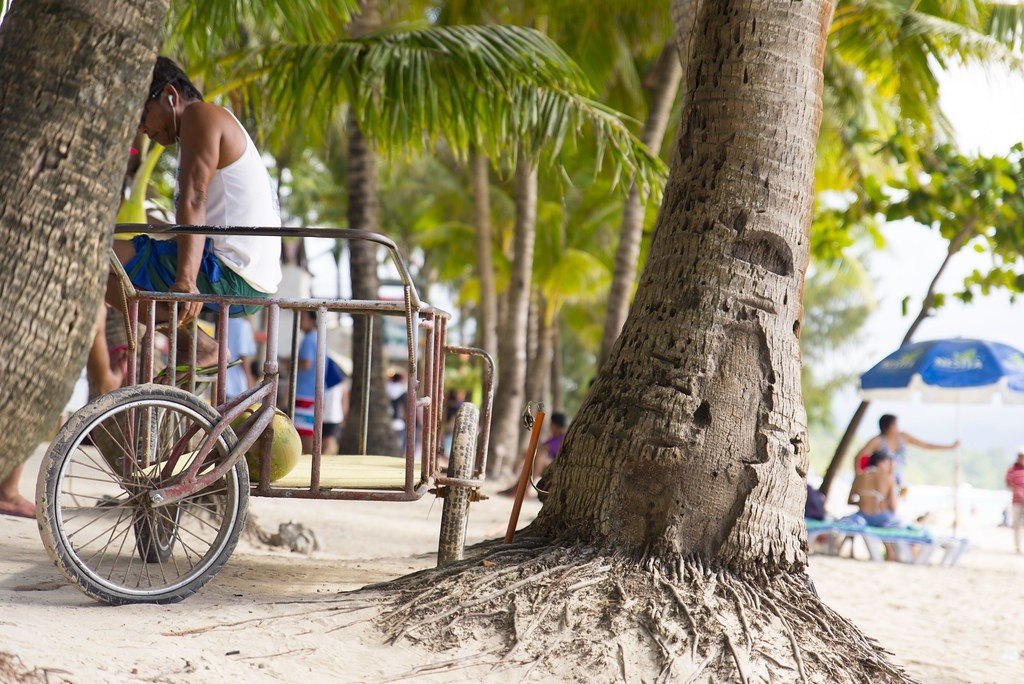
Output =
[{"x1": 855, "y1": 337, "x2": 1024, "y2": 533}]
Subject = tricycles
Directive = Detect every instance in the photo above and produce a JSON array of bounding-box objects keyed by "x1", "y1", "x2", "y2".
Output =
[{"x1": 35, "y1": 224, "x2": 495, "y2": 605}]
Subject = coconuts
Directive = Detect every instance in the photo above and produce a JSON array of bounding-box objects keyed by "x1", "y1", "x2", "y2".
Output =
[{"x1": 227, "y1": 405, "x2": 302, "y2": 480}]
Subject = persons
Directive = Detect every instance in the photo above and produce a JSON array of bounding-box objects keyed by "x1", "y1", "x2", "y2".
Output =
[
  {"x1": 386, "y1": 371, "x2": 408, "y2": 449},
  {"x1": 106, "y1": 55, "x2": 282, "y2": 386},
  {"x1": 226, "y1": 317, "x2": 257, "y2": 400},
  {"x1": 287, "y1": 309, "x2": 318, "y2": 456},
  {"x1": 1, "y1": 307, "x2": 125, "y2": 517},
  {"x1": 855, "y1": 415, "x2": 958, "y2": 488},
  {"x1": 1006, "y1": 451, "x2": 1024, "y2": 555},
  {"x1": 838, "y1": 451, "x2": 903, "y2": 560},
  {"x1": 499, "y1": 411, "x2": 567, "y2": 496},
  {"x1": 321, "y1": 358, "x2": 349, "y2": 455}
]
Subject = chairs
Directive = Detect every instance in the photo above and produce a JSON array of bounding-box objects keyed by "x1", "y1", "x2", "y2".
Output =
[{"x1": 803, "y1": 516, "x2": 968, "y2": 567}]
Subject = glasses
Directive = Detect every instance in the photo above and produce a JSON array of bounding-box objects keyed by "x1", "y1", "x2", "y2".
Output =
[{"x1": 140, "y1": 76, "x2": 178, "y2": 125}]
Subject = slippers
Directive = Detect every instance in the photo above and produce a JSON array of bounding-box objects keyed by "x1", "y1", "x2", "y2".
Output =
[{"x1": 153, "y1": 356, "x2": 243, "y2": 387}]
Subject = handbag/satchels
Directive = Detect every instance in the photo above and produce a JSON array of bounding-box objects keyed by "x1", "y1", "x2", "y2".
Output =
[{"x1": 324, "y1": 355, "x2": 346, "y2": 390}]
